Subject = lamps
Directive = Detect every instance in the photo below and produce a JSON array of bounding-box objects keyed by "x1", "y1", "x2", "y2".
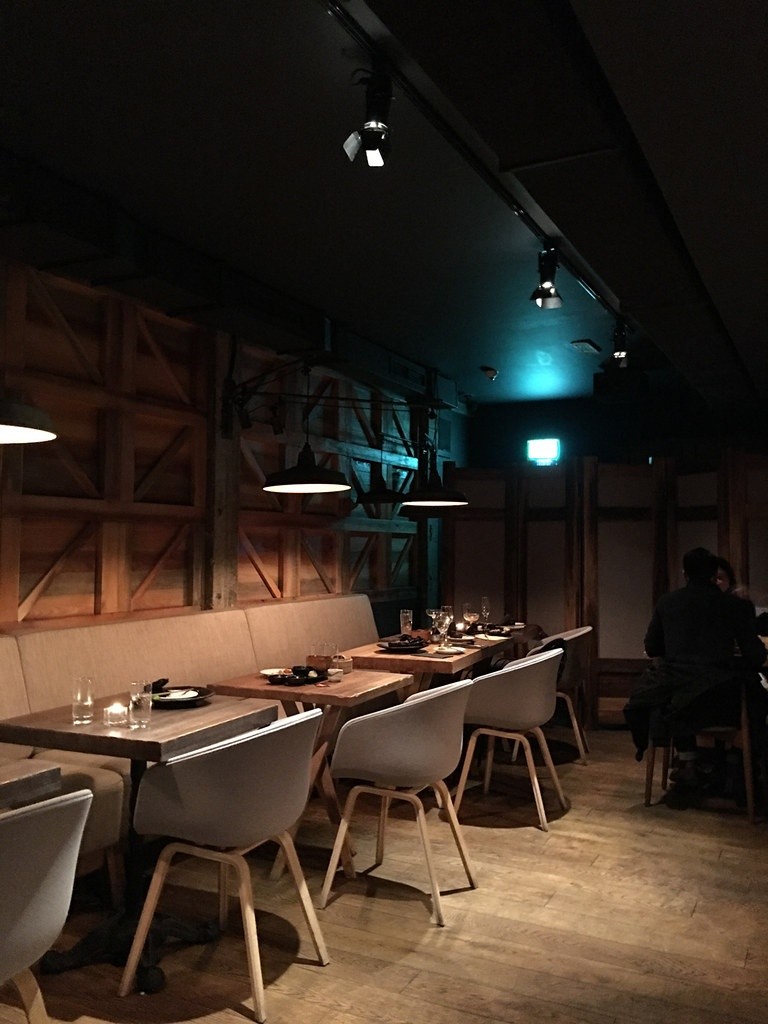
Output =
[
  {"x1": 597, "y1": 321, "x2": 635, "y2": 375},
  {"x1": 343, "y1": 65, "x2": 395, "y2": 168},
  {"x1": 219, "y1": 350, "x2": 472, "y2": 507},
  {"x1": 531, "y1": 250, "x2": 563, "y2": 310},
  {"x1": 0, "y1": 375, "x2": 59, "y2": 445}
]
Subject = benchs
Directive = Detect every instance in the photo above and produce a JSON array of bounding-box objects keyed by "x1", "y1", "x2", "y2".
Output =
[{"x1": 0, "y1": 592, "x2": 406, "y2": 910}]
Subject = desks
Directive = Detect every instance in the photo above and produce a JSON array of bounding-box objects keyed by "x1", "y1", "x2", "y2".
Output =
[
  {"x1": 207, "y1": 666, "x2": 414, "y2": 879},
  {"x1": 417, "y1": 623, "x2": 541, "y2": 659},
  {"x1": 1, "y1": 687, "x2": 278, "y2": 993},
  {"x1": 332, "y1": 635, "x2": 514, "y2": 809},
  {"x1": 0, "y1": 742, "x2": 63, "y2": 807}
]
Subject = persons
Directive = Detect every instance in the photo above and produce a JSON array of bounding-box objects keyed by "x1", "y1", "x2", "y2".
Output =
[
  {"x1": 644, "y1": 547, "x2": 768, "y2": 774},
  {"x1": 714, "y1": 555, "x2": 768, "y2": 648}
]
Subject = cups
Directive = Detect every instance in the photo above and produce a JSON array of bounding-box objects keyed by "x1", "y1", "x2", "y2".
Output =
[
  {"x1": 327, "y1": 668, "x2": 343, "y2": 682},
  {"x1": 127, "y1": 680, "x2": 153, "y2": 729},
  {"x1": 70, "y1": 677, "x2": 95, "y2": 724},
  {"x1": 399, "y1": 609, "x2": 413, "y2": 637},
  {"x1": 331, "y1": 657, "x2": 354, "y2": 673},
  {"x1": 102, "y1": 706, "x2": 128, "y2": 727}
]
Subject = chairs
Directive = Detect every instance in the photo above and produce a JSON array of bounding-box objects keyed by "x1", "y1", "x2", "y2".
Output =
[
  {"x1": 0, "y1": 789, "x2": 94, "y2": 1024},
  {"x1": 316, "y1": 626, "x2": 595, "y2": 925},
  {"x1": 118, "y1": 708, "x2": 329, "y2": 1024},
  {"x1": 644, "y1": 655, "x2": 757, "y2": 824}
]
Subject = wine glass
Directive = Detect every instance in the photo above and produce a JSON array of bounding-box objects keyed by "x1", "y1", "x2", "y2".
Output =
[{"x1": 426, "y1": 596, "x2": 491, "y2": 646}]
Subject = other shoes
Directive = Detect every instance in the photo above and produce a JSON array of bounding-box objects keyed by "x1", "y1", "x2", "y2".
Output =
[{"x1": 670, "y1": 766, "x2": 704, "y2": 786}]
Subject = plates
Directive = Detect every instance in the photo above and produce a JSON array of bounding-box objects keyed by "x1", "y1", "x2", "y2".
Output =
[
  {"x1": 435, "y1": 646, "x2": 466, "y2": 654},
  {"x1": 259, "y1": 668, "x2": 294, "y2": 677},
  {"x1": 148, "y1": 685, "x2": 216, "y2": 706},
  {"x1": 376, "y1": 641, "x2": 430, "y2": 654}
]
